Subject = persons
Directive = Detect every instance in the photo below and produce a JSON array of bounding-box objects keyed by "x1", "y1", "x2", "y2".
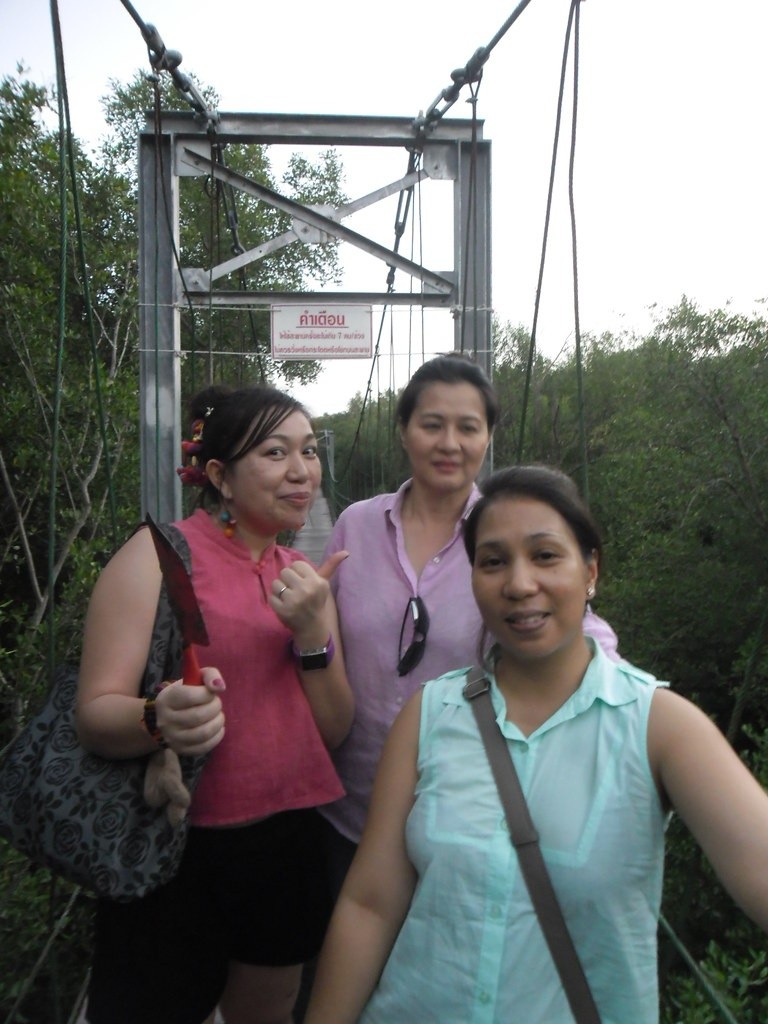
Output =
[
  {"x1": 319, "y1": 355, "x2": 768, "y2": 1024},
  {"x1": 76, "y1": 385, "x2": 353, "y2": 1024}
]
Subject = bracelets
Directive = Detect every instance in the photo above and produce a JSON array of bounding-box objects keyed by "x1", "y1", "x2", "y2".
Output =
[{"x1": 142, "y1": 680, "x2": 173, "y2": 749}]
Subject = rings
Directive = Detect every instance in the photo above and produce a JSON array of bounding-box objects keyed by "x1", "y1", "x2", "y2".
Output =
[{"x1": 277, "y1": 586, "x2": 288, "y2": 597}]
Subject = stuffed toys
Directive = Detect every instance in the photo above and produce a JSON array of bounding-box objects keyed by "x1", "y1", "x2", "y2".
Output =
[{"x1": 138, "y1": 749, "x2": 191, "y2": 823}]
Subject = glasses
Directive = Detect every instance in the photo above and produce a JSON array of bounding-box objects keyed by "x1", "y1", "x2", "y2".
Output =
[{"x1": 396, "y1": 594, "x2": 431, "y2": 677}]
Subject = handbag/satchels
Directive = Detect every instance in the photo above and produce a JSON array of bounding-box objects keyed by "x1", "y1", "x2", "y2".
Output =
[{"x1": 0, "y1": 519, "x2": 194, "y2": 904}]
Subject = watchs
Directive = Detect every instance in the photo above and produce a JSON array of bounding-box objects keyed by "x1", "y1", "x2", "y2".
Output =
[{"x1": 291, "y1": 634, "x2": 335, "y2": 672}]
